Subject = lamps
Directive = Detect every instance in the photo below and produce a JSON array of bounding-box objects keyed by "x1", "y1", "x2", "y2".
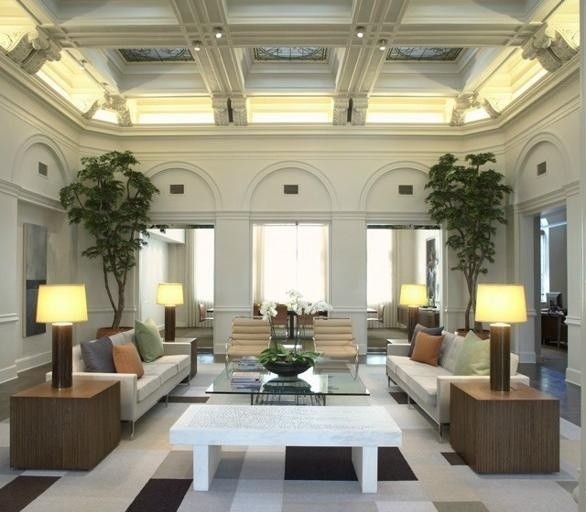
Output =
[
  {"x1": 312, "y1": 316, "x2": 359, "y2": 380},
  {"x1": 225, "y1": 316, "x2": 272, "y2": 377},
  {"x1": 198, "y1": 303, "x2": 388, "y2": 339}
]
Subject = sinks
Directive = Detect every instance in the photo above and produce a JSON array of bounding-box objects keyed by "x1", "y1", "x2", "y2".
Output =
[
  {"x1": 387, "y1": 331, "x2": 520, "y2": 440},
  {"x1": 46, "y1": 327, "x2": 191, "y2": 438}
]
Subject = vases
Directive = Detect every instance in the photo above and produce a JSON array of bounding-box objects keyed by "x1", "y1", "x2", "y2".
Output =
[{"x1": 253, "y1": 289, "x2": 331, "y2": 368}]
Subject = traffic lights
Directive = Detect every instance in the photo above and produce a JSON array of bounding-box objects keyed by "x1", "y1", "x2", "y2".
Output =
[
  {"x1": 78, "y1": 337, "x2": 115, "y2": 372},
  {"x1": 410, "y1": 331, "x2": 445, "y2": 366},
  {"x1": 112, "y1": 342, "x2": 144, "y2": 379},
  {"x1": 134, "y1": 318, "x2": 166, "y2": 362},
  {"x1": 455, "y1": 331, "x2": 491, "y2": 376},
  {"x1": 407, "y1": 324, "x2": 445, "y2": 355}
]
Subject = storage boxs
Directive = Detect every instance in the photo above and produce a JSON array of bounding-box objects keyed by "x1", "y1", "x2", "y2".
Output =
[{"x1": 170, "y1": 402, "x2": 401, "y2": 492}]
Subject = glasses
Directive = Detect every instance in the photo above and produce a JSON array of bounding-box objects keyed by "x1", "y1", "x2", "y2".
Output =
[
  {"x1": 474, "y1": 283, "x2": 528, "y2": 390},
  {"x1": 213, "y1": 27, "x2": 223, "y2": 38},
  {"x1": 193, "y1": 40, "x2": 202, "y2": 51},
  {"x1": 154, "y1": 282, "x2": 184, "y2": 341},
  {"x1": 400, "y1": 285, "x2": 427, "y2": 342},
  {"x1": 379, "y1": 40, "x2": 387, "y2": 50},
  {"x1": 36, "y1": 284, "x2": 88, "y2": 388},
  {"x1": 356, "y1": 27, "x2": 364, "y2": 38}
]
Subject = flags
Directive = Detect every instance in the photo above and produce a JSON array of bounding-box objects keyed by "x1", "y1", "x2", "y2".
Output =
[
  {"x1": 259, "y1": 355, "x2": 312, "y2": 376},
  {"x1": 263, "y1": 373, "x2": 311, "y2": 391}
]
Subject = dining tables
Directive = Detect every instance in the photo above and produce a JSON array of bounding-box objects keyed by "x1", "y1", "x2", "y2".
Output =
[
  {"x1": 60, "y1": 149, "x2": 160, "y2": 337},
  {"x1": 424, "y1": 154, "x2": 515, "y2": 340}
]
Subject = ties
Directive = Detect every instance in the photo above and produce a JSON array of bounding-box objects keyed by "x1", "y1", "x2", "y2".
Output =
[{"x1": 546, "y1": 292, "x2": 563, "y2": 312}]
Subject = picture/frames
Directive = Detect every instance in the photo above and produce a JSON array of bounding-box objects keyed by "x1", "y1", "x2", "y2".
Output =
[{"x1": 541, "y1": 309, "x2": 567, "y2": 344}]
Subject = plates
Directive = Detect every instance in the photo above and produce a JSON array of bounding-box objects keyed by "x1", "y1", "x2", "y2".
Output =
[
  {"x1": 10, "y1": 380, "x2": 122, "y2": 471},
  {"x1": 397, "y1": 306, "x2": 439, "y2": 327},
  {"x1": 160, "y1": 337, "x2": 197, "y2": 382},
  {"x1": 449, "y1": 381, "x2": 561, "y2": 473},
  {"x1": 387, "y1": 339, "x2": 410, "y2": 385}
]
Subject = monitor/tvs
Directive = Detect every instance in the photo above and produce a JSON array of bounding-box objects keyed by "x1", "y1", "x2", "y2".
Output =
[
  {"x1": 230, "y1": 383, "x2": 261, "y2": 390},
  {"x1": 229, "y1": 372, "x2": 262, "y2": 383},
  {"x1": 231, "y1": 354, "x2": 268, "y2": 371}
]
